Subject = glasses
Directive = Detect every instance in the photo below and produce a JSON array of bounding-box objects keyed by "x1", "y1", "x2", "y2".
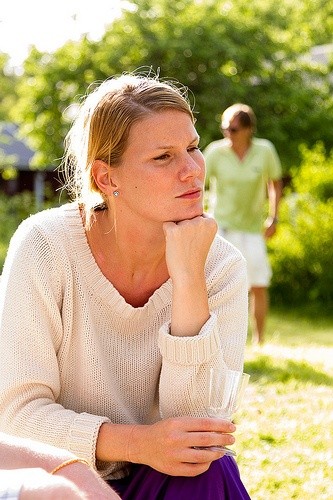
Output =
[{"x1": 229, "y1": 128, "x2": 238, "y2": 134}]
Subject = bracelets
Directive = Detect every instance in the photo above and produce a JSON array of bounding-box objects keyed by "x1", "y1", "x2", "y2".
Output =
[{"x1": 267, "y1": 217, "x2": 280, "y2": 223}]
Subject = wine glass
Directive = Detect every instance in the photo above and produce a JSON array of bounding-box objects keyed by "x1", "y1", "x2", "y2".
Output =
[{"x1": 193, "y1": 368, "x2": 250, "y2": 456}]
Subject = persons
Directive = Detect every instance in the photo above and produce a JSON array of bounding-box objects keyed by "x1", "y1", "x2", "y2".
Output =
[
  {"x1": 202, "y1": 102, "x2": 282, "y2": 347},
  {"x1": 0, "y1": 75, "x2": 251, "y2": 500}
]
